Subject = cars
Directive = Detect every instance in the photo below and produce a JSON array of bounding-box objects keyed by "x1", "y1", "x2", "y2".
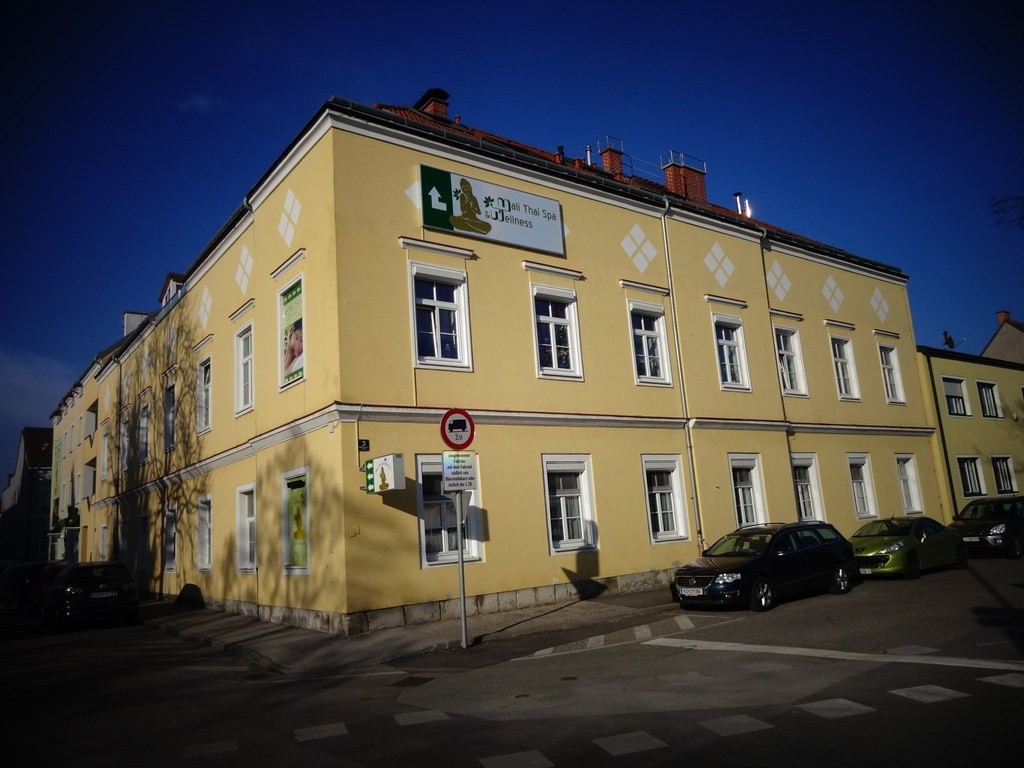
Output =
[
  {"x1": 846, "y1": 516, "x2": 967, "y2": 581},
  {"x1": 0, "y1": 559, "x2": 72, "y2": 613},
  {"x1": 47, "y1": 563, "x2": 145, "y2": 627}
]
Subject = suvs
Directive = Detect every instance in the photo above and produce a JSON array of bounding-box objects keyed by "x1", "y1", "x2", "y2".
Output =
[
  {"x1": 671, "y1": 520, "x2": 857, "y2": 612},
  {"x1": 946, "y1": 495, "x2": 1024, "y2": 560}
]
